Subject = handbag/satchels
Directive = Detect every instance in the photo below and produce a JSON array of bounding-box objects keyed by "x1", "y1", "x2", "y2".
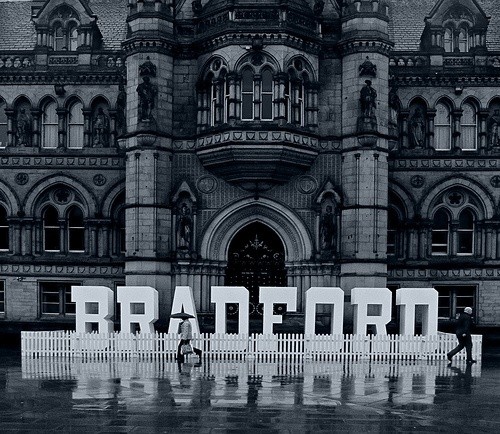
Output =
[{"x1": 180, "y1": 341, "x2": 194, "y2": 355}]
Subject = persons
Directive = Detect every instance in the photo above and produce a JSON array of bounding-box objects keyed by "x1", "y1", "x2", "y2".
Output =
[
  {"x1": 321, "y1": 205, "x2": 335, "y2": 249},
  {"x1": 136, "y1": 76, "x2": 159, "y2": 121},
  {"x1": 489, "y1": 109, "x2": 500, "y2": 147},
  {"x1": 92, "y1": 106, "x2": 108, "y2": 148},
  {"x1": 16, "y1": 106, "x2": 32, "y2": 146},
  {"x1": 410, "y1": 105, "x2": 425, "y2": 147},
  {"x1": 176, "y1": 317, "x2": 203, "y2": 361},
  {"x1": 446, "y1": 306, "x2": 477, "y2": 363},
  {"x1": 360, "y1": 79, "x2": 378, "y2": 118},
  {"x1": 176, "y1": 203, "x2": 193, "y2": 251}
]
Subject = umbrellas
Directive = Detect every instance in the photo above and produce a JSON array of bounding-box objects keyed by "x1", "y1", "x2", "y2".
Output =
[{"x1": 169, "y1": 313, "x2": 195, "y2": 319}]
